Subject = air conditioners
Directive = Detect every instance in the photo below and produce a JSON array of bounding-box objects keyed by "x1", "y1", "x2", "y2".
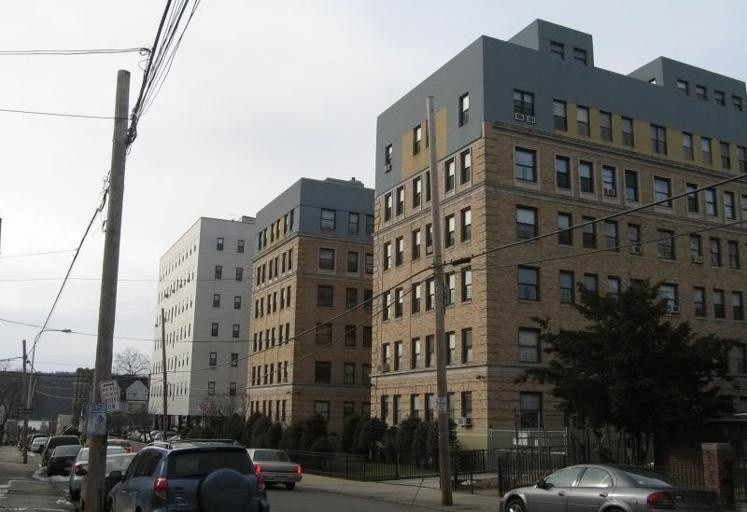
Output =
[
  {"x1": 629, "y1": 244, "x2": 642, "y2": 255},
  {"x1": 376, "y1": 363, "x2": 390, "y2": 374},
  {"x1": 384, "y1": 163, "x2": 392, "y2": 172},
  {"x1": 514, "y1": 113, "x2": 524, "y2": 121},
  {"x1": 605, "y1": 188, "x2": 616, "y2": 196},
  {"x1": 670, "y1": 305, "x2": 680, "y2": 315},
  {"x1": 525, "y1": 114, "x2": 535, "y2": 124},
  {"x1": 455, "y1": 415, "x2": 472, "y2": 427},
  {"x1": 692, "y1": 255, "x2": 705, "y2": 265}
]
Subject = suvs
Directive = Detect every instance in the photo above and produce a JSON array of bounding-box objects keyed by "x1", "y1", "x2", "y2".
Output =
[
  {"x1": 104, "y1": 439, "x2": 269, "y2": 512},
  {"x1": 41, "y1": 434, "x2": 80, "y2": 470}
]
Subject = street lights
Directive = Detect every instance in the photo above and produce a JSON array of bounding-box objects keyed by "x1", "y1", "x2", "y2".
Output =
[{"x1": 25, "y1": 328, "x2": 71, "y2": 423}]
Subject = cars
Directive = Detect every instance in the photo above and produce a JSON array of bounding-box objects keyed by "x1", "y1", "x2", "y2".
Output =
[
  {"x1": 68, "y1": 445, "x2": 129, "y2": 499},
  {"x1": 128, "y1": 428, "x2": 181, "y2": 444},
  {"x1": 498, "y1": 463, "x2": 719, "y2": 512},
  {"x1": 26, "y1": 433, "x2": 49, "y2": 454},
  {"x1": 46, "y1": 445, "x2": 83, "y2": 478},
  {"x1": 105, "y1": 452, "x2": 137, "y2": 495},
  {"x1": 245, "y1": 447, "x2": 303, "y2": 490}
]
